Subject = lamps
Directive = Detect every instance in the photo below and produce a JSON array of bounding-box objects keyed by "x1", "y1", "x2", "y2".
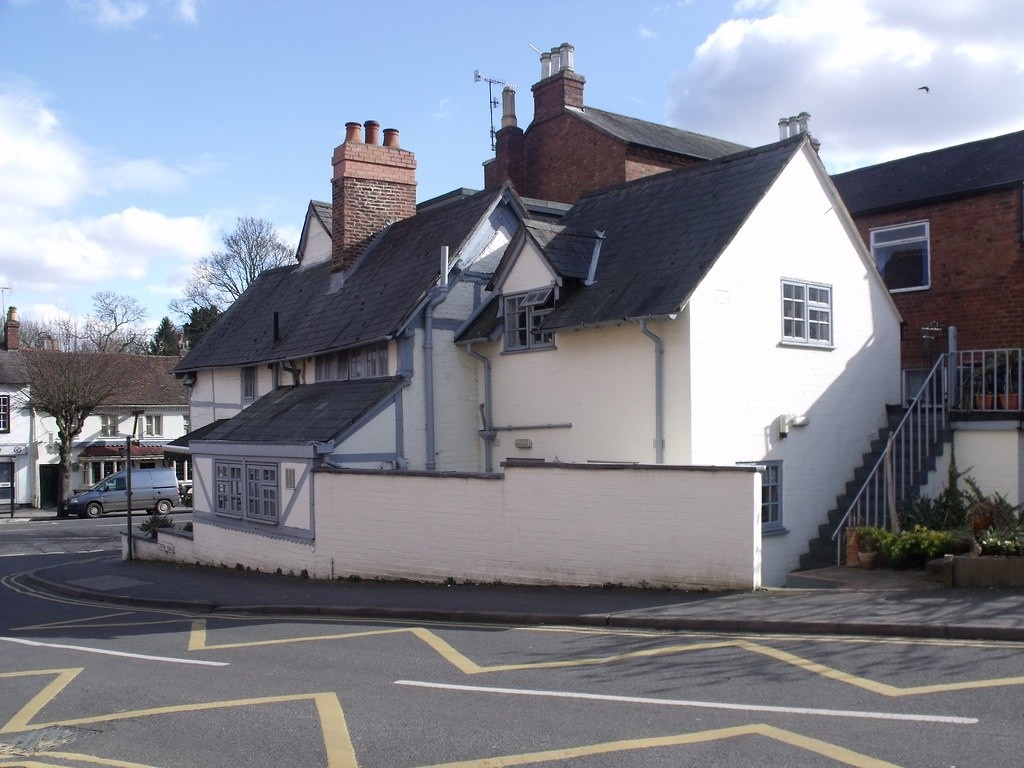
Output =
[
  {"x1": 183, "y1": 377, "x2": 197, "y2": 387},
  {"x1": 779, "y1": 415, "x2": 789, "y2": 438}
]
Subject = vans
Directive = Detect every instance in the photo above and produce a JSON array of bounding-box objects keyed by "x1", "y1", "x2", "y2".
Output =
[{"x1": 63, "y1": 467, "x2": 180, "y2": 519}]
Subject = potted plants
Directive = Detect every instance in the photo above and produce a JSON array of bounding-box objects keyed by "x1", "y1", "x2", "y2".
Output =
[
  {"x1": 966, "y1": 342, "x2": 1024, "y2": 410},
  {"x1": 843, "y1": 494, "x2": 973, "y2": 570},
  {"x1": 136, "y1": 511, "x2": 176, "y2": 539}
]
